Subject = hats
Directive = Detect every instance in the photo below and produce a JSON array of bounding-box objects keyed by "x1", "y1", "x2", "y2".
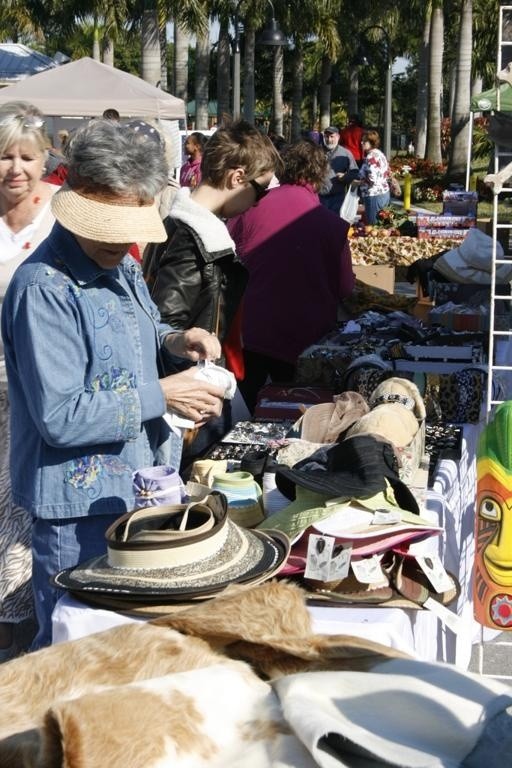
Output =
[
  {"x1": 49, "y1": 432, "x2": 460, "y2": 619},
  {"x1": 51, "y1": 166, "x2": 168, "y2": 244},
  {"x1": 324, "y1": 126, "x2": 338, "y2": 134},
  {"x1": 431, "y1": 228, "x2": 512, "y2": 287},
  {"x1": 299, "y1": 376, "x2": 461, "y2": 447}
]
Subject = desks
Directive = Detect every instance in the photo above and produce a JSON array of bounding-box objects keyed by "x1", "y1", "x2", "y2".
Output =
[{"x1": 46, "y1": 419, "x2": 486, "y2": 687}]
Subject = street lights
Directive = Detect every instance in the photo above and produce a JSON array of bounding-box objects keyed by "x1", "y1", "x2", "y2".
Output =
[
  {"x1": 350, "y1": 21, "x2": 395, "y2": 166},
  {"x1": 227, "y1": 0, "x2": 291, "y2": 120}
]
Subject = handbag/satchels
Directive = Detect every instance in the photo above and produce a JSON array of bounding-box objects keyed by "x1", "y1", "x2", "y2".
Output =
[{"x1": 388, "y1": 177, "x2": 401, "y2": 196}]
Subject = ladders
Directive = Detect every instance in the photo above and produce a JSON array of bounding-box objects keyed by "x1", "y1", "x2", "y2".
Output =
[{"x1": 487, "y1": 5, "x2": 512, "y2": 433}]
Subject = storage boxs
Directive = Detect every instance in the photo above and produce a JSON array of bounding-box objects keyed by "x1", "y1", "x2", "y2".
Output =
[{"x1": 349, "y1": 263, "x2": 397, "y2": 293}]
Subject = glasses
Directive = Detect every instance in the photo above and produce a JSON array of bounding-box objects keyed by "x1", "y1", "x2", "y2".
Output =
[{"x1": 228, "y1": 165, "x2": 270, "y2": 201}]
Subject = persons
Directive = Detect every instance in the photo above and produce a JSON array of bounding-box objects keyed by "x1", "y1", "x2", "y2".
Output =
[{"x1": 1, "y1": 100, "x2": 401, "y2": 653}]
